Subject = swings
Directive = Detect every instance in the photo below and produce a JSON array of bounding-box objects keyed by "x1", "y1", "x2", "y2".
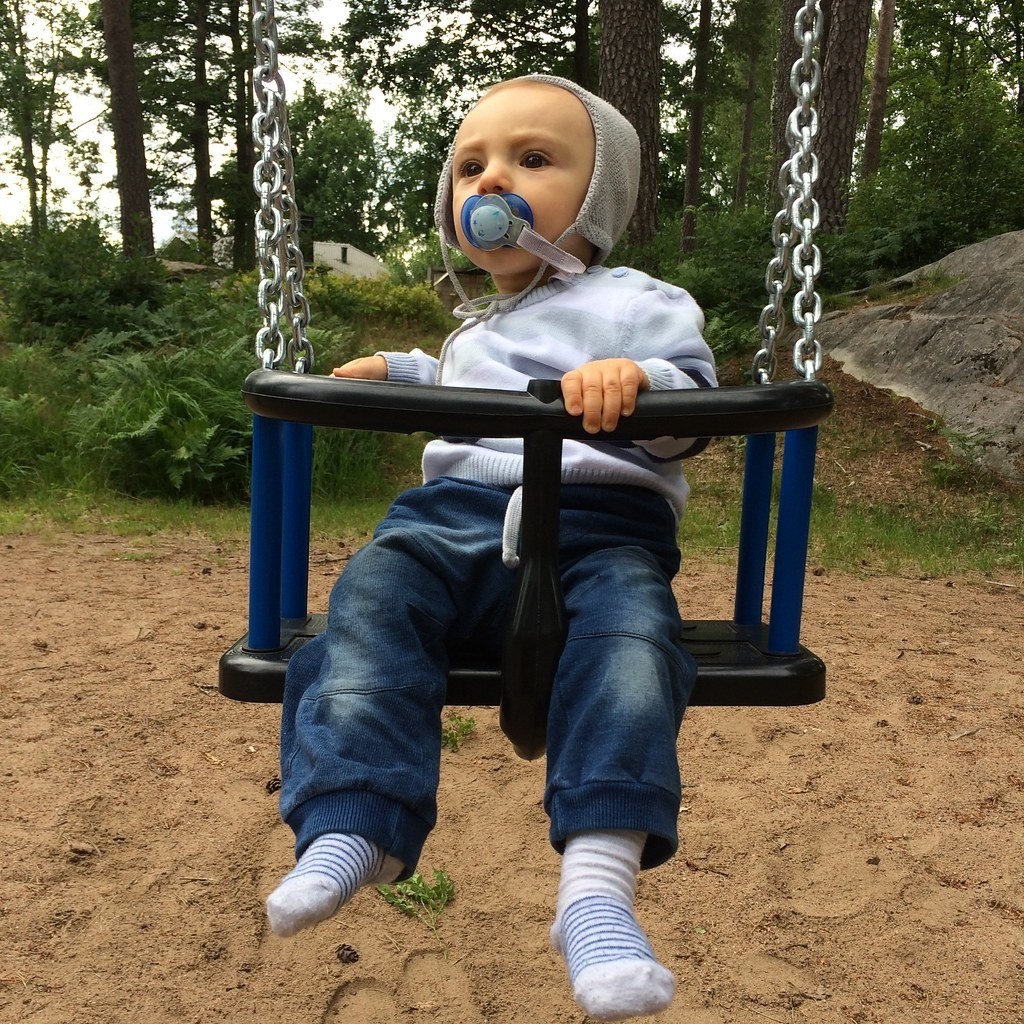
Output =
[{"x1": 207, "y1": 1, "x2": 832, "y2": 710}]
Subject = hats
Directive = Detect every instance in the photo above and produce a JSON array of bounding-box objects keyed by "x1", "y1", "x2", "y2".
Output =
[{"x1": 433, "y1": 75, "x2": 641, "y2": 330}]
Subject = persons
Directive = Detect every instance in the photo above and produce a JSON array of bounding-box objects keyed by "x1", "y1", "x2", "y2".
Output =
[{"x1": 265, "y1": 75, "x2": 717, "y2": 1018}]
